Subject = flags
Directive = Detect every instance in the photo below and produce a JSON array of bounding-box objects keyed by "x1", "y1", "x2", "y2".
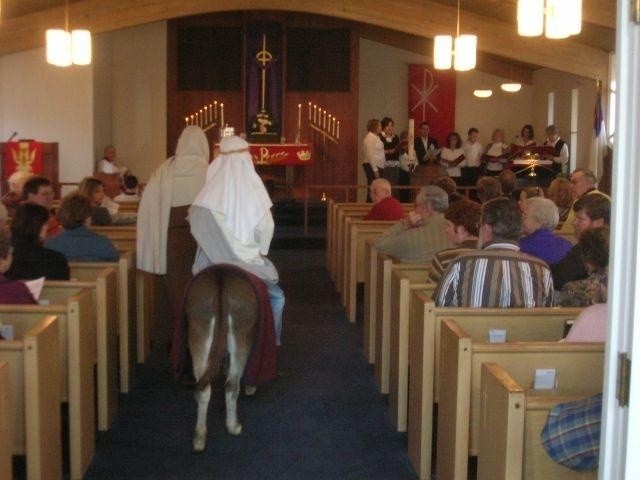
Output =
[{"x1": 589, "y1": 92, "x2": 608, "y2": 185}]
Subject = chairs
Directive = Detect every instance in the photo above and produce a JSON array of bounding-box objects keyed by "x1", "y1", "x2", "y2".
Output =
[
  {"x1": 0, "y1": 201, "x2": 155, "y2": 480},
  {"x1": 324, "y1": 196, "x2": 608, "y2": 480}
]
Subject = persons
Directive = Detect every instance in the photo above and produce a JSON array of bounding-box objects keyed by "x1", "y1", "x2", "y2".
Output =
[
  {"x1": 363, "y1": 168, "x2": 611, "y2": 342},
  {"x1": 185, "y1": 136, "x2": 285, "y2": 346},
  {"x1": 362, "y1": 119, "x2": 570, "y2": 201},
  {"x1": 135, "y1": 125, "x2": 210, "y2": 276},
  {"x1": 0, "y1": 144, "x2": 142, "y2": 305},
  {"x1": 541, "y1": 392, "x2": 602, "y2": 470}
]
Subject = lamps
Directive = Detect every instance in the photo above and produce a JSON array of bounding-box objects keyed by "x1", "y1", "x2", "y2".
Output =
[
  {"x1": 473, "y1": 71, "x2": 493, "y2": 99},
  {"x1": 44, "y1": 1, "x2": 92, "y2": 67},
  {"x1": 516, "y1": 0, "x2": 582, "y2": 40},
  {"x1": 500, "y1": 63, "x2": 521, "y2": 94},
  {"x1": 433, "y1": 0, "x2": 478, "y2": 71}
]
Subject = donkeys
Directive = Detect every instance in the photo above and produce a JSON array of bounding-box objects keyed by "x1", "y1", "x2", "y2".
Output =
[{"x1": 182, "y1": 263, "x2": 260, "y2": 454}]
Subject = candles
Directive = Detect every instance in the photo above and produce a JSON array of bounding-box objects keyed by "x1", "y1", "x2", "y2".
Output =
[
  {"x1": 184, "y1": 101, "x2": 224, "y2": 129},
  {"x1": 298, "y1": 102, "x2": 340, "y2": 139}
]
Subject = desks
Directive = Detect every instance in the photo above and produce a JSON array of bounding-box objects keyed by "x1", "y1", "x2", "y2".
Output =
[{"x1": 212, "y1": 143, "x2": 315, "y2": 238}]
